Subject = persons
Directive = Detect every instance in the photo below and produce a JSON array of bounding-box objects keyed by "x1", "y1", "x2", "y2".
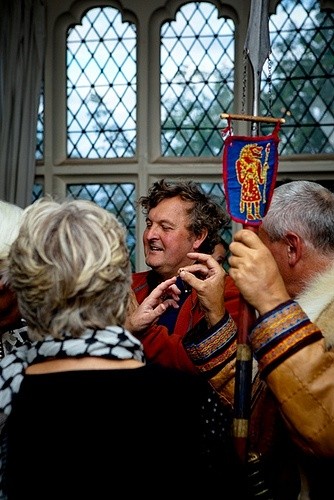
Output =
[
  {"x1": 0, "y1": 193, "x2": 238, "y2": 500},
  {"x1": 178, "y1": 181, "x2": 334, "y2": 500},
  {"x1": 131, "y1": 178, "x2": 288, "y2": 500}
]
